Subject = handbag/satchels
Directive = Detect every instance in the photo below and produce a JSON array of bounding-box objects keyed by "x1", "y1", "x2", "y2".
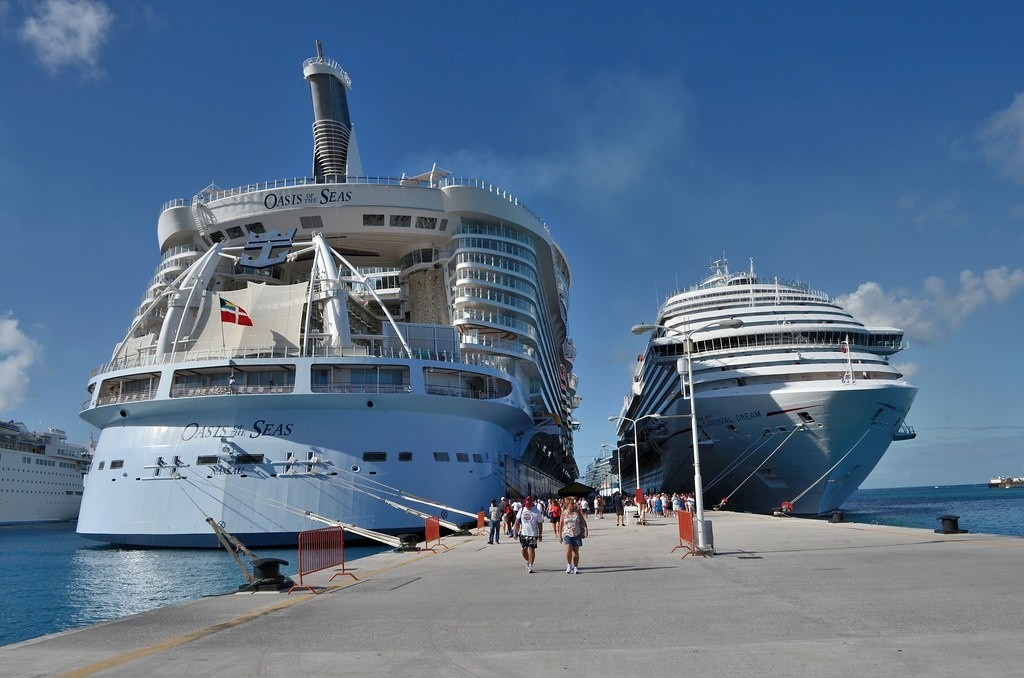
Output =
[
  {"x1": 506, "y1": 506, "x2": 514, "y2": 519},
  {"x1": 517, "y1": 507, "x2": 524, "y2": 524},
  {"x1": 577, "y1": 510, "x2": 585, "y2": 538}
]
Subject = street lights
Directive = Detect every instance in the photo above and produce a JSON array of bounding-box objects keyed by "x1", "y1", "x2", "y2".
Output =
[
  {"x1": 608, "y1": 413, "x2": 661, "y2": 525},
  {"x1": 631, "y1": 319, "x2": 744, "y2": 554},
  {"x1": 602, "y1": 443, "x2": 636, "y2": 496}
]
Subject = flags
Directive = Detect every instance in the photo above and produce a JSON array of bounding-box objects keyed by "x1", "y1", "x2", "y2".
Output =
[{"x1": 219, "y1": 297, "x2": 254, "y2": 327}]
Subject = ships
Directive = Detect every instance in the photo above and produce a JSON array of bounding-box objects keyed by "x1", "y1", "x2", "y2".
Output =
[
  {"x1": 608, "y1": 252, "x2": 921, "y2": 517},
  {"x1": 73, "y1": 37, "x2": 586, "y2": 550}
]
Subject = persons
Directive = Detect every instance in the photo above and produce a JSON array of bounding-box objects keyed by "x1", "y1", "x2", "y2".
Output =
[
  {"x1": 559, "y1": 497, "x2": 588, "y2": 574},
  {"x1": 487, "y1": 493, "x2": 696, "y2": 545},
  {"x1": 514, "y1": 498, "x2": 543, "y2": 573},
  {"x1": 227, "y1": 376, "x2": 235, "y2": 394},
  {"x1": 841, "y1": 345, "x2": 847, "y2": 353},
  {"x1": 269, "y1": 378, "x2": 275, "y2": 392}
]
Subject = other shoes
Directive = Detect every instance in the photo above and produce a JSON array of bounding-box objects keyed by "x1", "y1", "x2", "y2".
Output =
[
  {"x1": 526, "y1": 563, "x2": 533, "y2": 573},
  {"x1": 574, "y1": 568, "x2": 578, "y2": 574},
  {"x1": 566, "y1": 565, "x2": 572, "y2": 574},
  {"x1": 488, "y1": 542, "x2": 493, "y2": 544}
]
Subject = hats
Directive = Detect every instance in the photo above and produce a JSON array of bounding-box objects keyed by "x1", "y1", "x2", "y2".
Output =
[
  {"x1": 501, "y1": 497, "x2": 505, "y2": 500},
  {"x1": 524, "y1": 498, "x2": 532, "y2": 508}
]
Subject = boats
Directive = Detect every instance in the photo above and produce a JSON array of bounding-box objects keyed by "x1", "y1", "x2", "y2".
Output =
[
  {"x1": 987, "y1": 477, "x2": 1024, "y2": 489},
  {"x1": 0, "y1": 420, "x2": 97, "y2": 529}
]
